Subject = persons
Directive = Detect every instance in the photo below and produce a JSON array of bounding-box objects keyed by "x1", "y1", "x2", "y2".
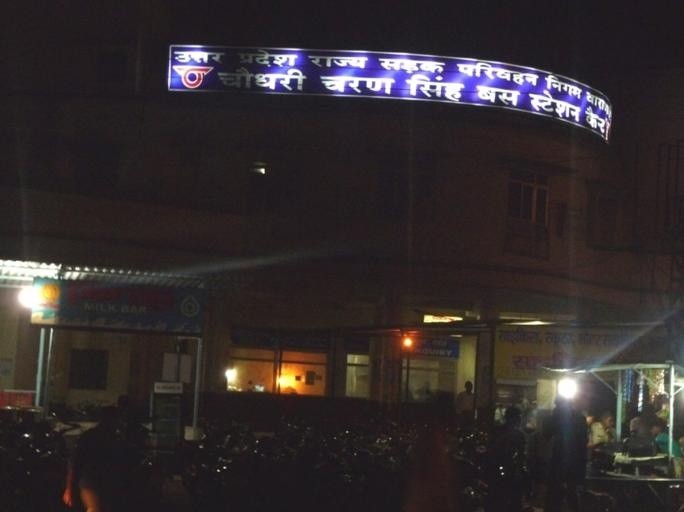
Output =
[
  {"x1": 480, "y1": 389, "x2": 683, "y2": 512},
  {"x1": 457, "y1": 380, "x2": 474, "y2": 421},
  {"x1": 61, "y1": 429, "x2": 110, "y2": 511}
]
